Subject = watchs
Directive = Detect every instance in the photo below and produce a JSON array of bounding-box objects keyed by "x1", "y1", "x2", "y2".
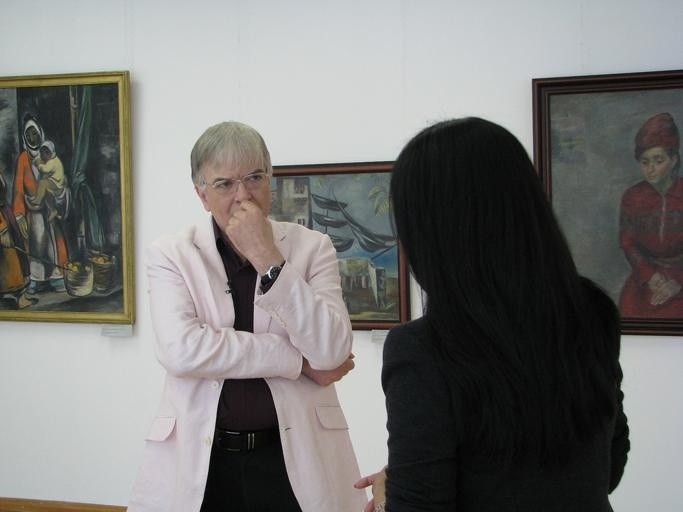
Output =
[{"x1": 260, "y1": 261, "x2": 286, "y2": 286}]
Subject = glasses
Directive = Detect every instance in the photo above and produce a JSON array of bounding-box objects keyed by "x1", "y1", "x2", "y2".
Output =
[{"x1": 199, "y1": 172, "x2": 271, "y2": 195}]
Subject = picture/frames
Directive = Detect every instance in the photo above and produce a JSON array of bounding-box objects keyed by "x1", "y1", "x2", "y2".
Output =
[
  {"x1": 529, "y1": 68, "x2": 682, "y2": 338},
  {"x1": 0, "y1": 69, "x2": 138, "y2": 328},
  {"x1": 266, "y1": 160, "x2": 412, "y2": 332}
]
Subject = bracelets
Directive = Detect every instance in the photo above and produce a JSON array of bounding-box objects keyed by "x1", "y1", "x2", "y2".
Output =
[{"x1": 374, "y1": 500, "x2": 386, "y2": 512}]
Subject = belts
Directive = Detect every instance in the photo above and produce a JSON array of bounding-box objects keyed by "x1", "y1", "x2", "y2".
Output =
[{"x1": 212, "y1": 429, "x2": 282, "y2": 454}]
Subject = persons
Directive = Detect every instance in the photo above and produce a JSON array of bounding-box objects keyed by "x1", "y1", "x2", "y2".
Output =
[
  {"x1": 126, "y1": 120, "x2": 369, "y2": 512},
  {"x1": 30, "y1": 141, "x2": 65, "y2": 223},
  {"x1": 619, "y1": 111, "x2": 683, "y2": 318},
  {"x1": 352, "y1": 116, "x2": 630, "y2": 511},
  {"x1": 1, "y1": 172, "x2": 39, "y2": 310},
  {"x1": 11, "y1": 119, "x2": 69, "y2": 295}
]
